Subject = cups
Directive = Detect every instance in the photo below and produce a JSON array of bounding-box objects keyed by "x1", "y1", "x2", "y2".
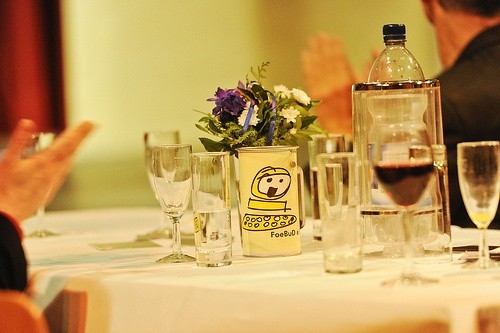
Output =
[
  {"x1": 353, "y1": 79, "x2": 452, "y2": 264},
  {"x1": 189, "y1": 152, "x2": 233, "y2": 267},
  {"x1": 309, "y1": 134, "x2": 346, "y2": 240},
  {"x1": 316, "y1": 152, "x2": 362, "y2": 273},
  {"x1": 235, "y1": 145, "x2": 301, "y2": 257}
]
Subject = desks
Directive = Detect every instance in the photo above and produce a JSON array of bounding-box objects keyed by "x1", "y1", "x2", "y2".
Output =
[{"x1": 15, "y1": 200, "x2": 499, "y2": 331}]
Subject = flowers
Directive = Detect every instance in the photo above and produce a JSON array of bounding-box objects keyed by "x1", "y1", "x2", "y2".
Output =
[{"x1": 197, "y1": 63, "x2": 325, "y2": 160}]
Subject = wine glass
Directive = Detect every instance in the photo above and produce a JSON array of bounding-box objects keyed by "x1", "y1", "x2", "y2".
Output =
[
  {"x1": 369, "y1": 122, "x2": 439, "y2": 286},
  {"x1": 152, "y1": 145, "x2": 197, "y2": 264},
  {"x1": 457, "y1": 141, "x2": 500, "y2": 271},
  {"x1": 25, "y1": 132, "x2": 60, "y2": 237},
  {"x1": 145, "y1": 130, "x2": 181, "y2": 246}
]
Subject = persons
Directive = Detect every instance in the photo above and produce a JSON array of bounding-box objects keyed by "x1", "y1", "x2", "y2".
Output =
[
  {"x1": 300, "y1": 0, "x2": 500, "y2": 231},
  {"x1": 0, "y1": 117, "x2": 92, "y2": 294}
]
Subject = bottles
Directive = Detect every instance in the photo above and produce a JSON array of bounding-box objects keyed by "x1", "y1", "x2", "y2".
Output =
[{"x1": 366, "y1": 24, "x2": 429, "y2": 144}]
